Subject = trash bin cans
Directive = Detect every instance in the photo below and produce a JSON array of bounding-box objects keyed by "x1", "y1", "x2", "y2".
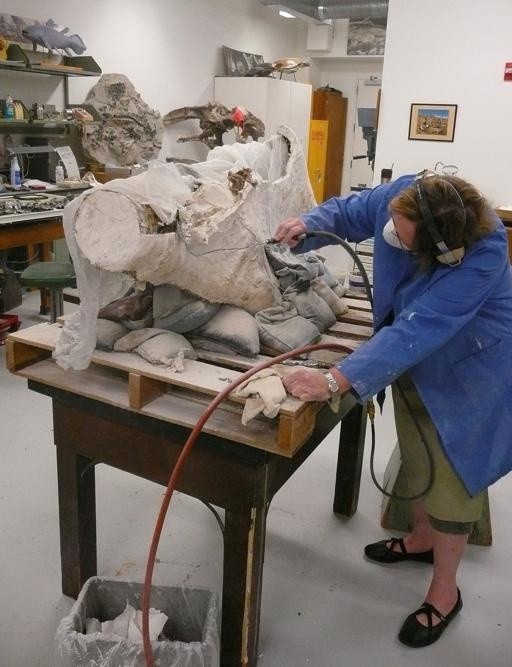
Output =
[{"x1": 60, "y1": 576, "x2": 220, "y2": 667}]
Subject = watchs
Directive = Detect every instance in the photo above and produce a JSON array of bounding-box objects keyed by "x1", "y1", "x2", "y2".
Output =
[{"x1": 322, "y1": 370, "x2": 341, "y2": 401}]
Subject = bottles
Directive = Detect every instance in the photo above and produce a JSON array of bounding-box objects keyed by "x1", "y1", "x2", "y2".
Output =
[
  {"x1": 6, "y1": 93, "x2": 15, "y2": 118},
  {"x1": 55, "y1": 165, "x2": 64, "y2": 183},
  {"x1": 10, "y1": 155, "x2": 21, "y2": 187}
]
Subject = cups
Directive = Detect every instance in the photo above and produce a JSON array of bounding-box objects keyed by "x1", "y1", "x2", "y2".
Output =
[{"x1": 381, "y1": 168, "x2": 392, "y2": 184}]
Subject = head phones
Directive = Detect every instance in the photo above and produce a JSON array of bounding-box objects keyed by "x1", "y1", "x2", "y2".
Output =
[{"x1": 412, "y1": 169, "x2": 465, "y2": 267}]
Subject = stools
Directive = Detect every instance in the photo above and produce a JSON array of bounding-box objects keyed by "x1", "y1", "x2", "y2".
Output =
[{"x1": 20, "y1": 262, "x2": 77, "y2": 323}]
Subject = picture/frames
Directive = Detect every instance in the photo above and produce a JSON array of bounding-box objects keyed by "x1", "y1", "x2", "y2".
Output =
[{"x1": 408, "y1": 102, "x2": 457, "y2": 142}]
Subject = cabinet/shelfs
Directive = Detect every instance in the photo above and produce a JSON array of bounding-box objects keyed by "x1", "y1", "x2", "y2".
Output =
[{"x1": 1, "y1": 45, "x2": 103, "y2": 134}]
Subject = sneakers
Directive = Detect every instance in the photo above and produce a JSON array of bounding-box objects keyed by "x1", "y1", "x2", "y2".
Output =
[
  {"x1": 364, "y1": 536, "x2": 433, "y2": 565},
  {"x1": 398, "y1": 584, "x2": 463, "y2": 647}
]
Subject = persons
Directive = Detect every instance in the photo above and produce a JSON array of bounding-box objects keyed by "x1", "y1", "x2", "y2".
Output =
[{"x1": 270, "y1": 168, "x2": 511, "y2": 648}]
